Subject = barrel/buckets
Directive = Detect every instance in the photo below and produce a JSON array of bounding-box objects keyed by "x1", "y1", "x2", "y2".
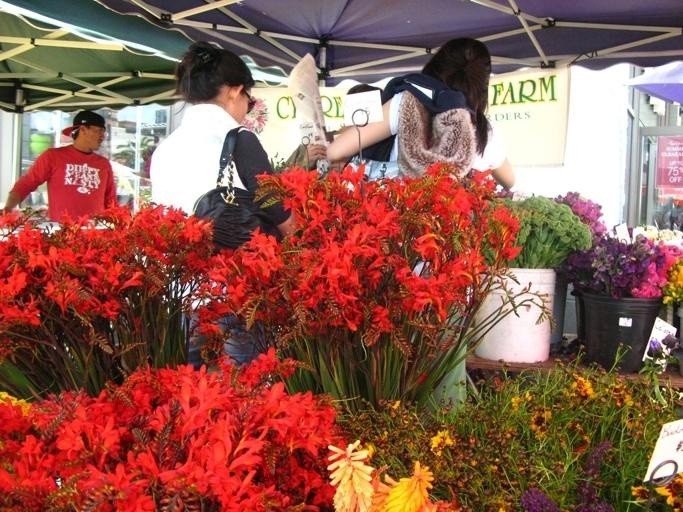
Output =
[{"x1": 474, "y1": 267, "x2": 555, "y2": 364}]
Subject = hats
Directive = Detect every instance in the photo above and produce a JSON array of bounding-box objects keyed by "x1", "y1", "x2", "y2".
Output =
[{"x1": 62, "y1": 112, "x2": 106, "y2": 136}]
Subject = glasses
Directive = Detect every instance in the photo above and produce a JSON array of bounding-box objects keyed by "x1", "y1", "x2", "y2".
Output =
[{"x1": 225, "y1": 80, "x2": 256, "y2": 114}]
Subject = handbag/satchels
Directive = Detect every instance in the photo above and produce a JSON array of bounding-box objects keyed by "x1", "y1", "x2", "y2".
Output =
[{"x1": 192, "y1": 126, "x2": 284, "y2": 250}]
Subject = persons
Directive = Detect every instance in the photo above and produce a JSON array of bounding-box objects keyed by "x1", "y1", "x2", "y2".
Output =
[
  {"x1": 0, "y1": 112, "x2": 119, "y2": 225},
  {"x1": 151, "y1": 42, "x2": 300, "y2": 373},
  {"x1": 327, "y1": 36, "x2": 516, "y2": 411},
  {"x1": 276, "y1": 82, "x2": 384, "y2": 175}
]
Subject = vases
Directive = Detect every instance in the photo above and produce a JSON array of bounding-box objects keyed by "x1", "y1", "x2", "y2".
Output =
[
  {"x1": 570, "y1": 291, "x2": 585, "y2": 343},
  {"x1": 550, "y1": 278, "x2": 567, "y2": 356}
]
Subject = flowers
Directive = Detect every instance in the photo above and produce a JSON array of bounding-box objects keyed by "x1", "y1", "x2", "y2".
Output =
[
  {"x1": 568, "y1": 226, "x2": 683, "y2": 304},
  {"x1": 554, "y1": 192, "x2": 606, "y2": 283}
]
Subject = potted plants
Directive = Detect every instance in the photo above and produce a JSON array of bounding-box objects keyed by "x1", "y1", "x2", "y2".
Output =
[{"x1": 476, "y1": 196, "x2": 592, "y2": 364}]
